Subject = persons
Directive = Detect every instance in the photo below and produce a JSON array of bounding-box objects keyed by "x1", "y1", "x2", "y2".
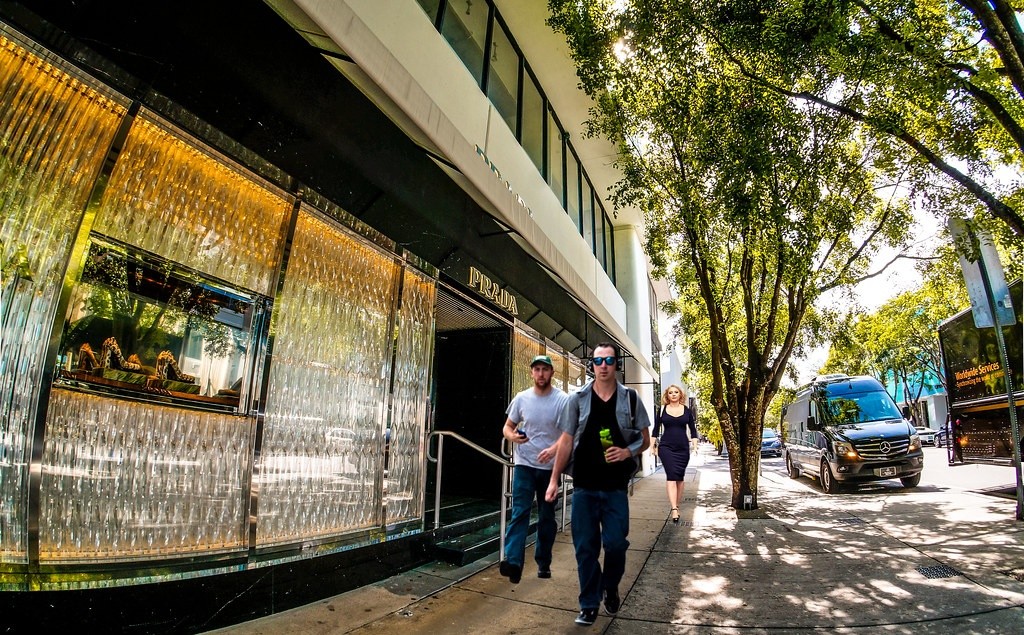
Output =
[
  {"x1": 499, "y1": 356, "x2": 570, "y2": 585},
  {"x1": 544, "y1": 342, "x2": 650, "y2": 625},
  {"x1": 649, "y1": 384, "x2": 699, "y2": 522}
]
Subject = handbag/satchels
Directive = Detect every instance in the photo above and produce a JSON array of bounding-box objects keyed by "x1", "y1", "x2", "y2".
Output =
[{"x1": 653, "y1": 434, "x2": 661, "y2": 456}]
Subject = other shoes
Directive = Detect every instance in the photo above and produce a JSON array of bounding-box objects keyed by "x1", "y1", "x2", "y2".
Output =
[
  {"x1": 499, "y1": 560, "x2": 522, "y2": 583},
  {"x1": 538, "y1": 566, "x2": 551, "y2": 578}
]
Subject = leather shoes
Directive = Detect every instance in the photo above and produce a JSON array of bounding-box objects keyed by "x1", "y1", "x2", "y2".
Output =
[{"x1": 671, "y1": 506, "x2": 680, "y2": 522}]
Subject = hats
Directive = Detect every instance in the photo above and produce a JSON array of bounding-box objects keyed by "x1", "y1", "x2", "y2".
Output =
[{"x1": 530, "y1": 356, "x2": 554, "y2": 370}]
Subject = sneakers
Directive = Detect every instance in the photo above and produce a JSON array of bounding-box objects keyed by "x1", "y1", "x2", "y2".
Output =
[
  {"x1": 574, "y1": 606, "x2": 599, "y2": 624},
  {"x1": 603, "y1": 585, "x2": 620, "y2": 615}
]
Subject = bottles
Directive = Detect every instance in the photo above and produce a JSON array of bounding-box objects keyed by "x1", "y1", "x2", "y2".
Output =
[{"x1": 599, "y1": 428, "x2": 614, "y2": 463}]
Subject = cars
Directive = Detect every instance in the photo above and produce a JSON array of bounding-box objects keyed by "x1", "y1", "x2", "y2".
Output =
[
  {"x1": 757, "y1": 425, "x2": 782, "y2": 458},
  {"x1": 914, "y1": 425, "x2": 937, "y2": 446},
  {"x1": 933, "y1": 421, "x2": 953, "y2": 449}
]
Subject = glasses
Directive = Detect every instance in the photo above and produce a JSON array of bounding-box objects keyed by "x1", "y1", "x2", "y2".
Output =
[{"x1": 592, "y1": 356, "x2": 617, "y2": 365}]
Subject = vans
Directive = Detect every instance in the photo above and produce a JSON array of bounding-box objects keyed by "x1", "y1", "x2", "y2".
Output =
[{"x1": 779, "y1": 373, "x2": 926, "y2": 491}]
